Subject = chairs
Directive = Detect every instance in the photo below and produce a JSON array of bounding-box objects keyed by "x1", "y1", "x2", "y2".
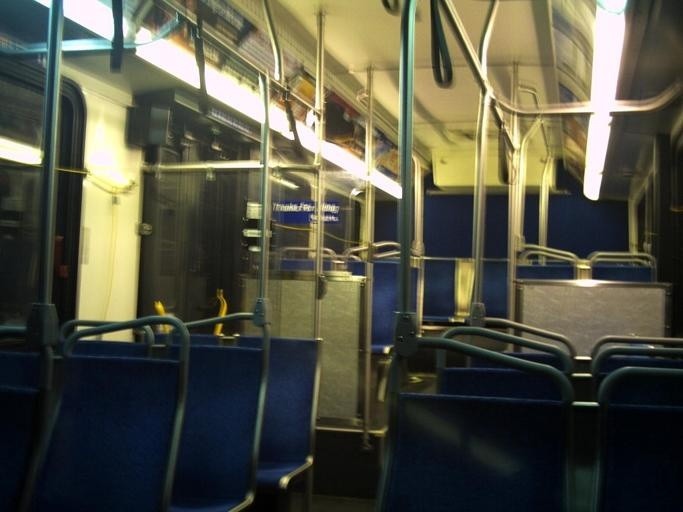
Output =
[
  {"x1": 411, "y1": 253, "x2": 516, "y2": 329},
  {"x1": 515, "y1": 236, "x2": 658, "y2": 282},
  {"x1": 282, "y1": 238, "x2": 419, "y2": 403}
]
